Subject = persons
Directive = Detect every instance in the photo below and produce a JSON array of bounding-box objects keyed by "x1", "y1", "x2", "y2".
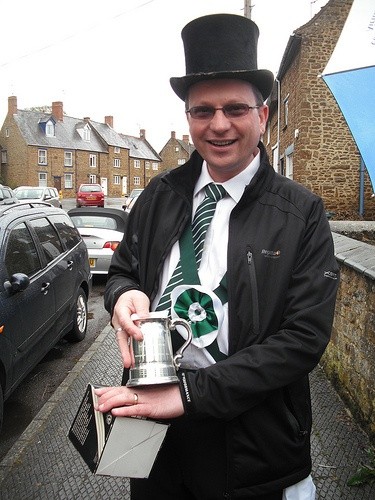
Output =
[
  {"x1": 92, "y1": 13, "x2": 341, "y2": 500},
  {"x1": 59, "y1": 191, "x2": 63, "y2": 209}
]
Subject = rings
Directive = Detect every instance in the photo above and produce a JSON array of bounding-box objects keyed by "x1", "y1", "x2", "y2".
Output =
[
  {"x1": 115, "y1": 328, "x2": 123, "y2": 335},
  {"x1": 134, "y1": 393, "x2": 138, "y2": 404}
]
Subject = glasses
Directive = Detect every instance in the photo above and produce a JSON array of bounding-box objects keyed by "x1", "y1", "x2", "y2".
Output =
[{"x1": 185, "y1": 102, "x2": 262, "y2": 120}]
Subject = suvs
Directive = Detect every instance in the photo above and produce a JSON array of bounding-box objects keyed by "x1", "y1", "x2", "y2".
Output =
[
  {"x1": 0, "y1": 183, "x2": 21, "y2": 206},
  {"x1": 0, "y1": 200, "x2": 95, "y2": 436}
]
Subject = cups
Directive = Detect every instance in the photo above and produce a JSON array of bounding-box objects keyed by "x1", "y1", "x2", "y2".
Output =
[{"x1": 126, "y1": 318, "x2": 193, "y2": 387}]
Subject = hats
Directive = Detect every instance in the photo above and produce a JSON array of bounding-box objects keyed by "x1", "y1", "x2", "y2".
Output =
[{"x1": 169, "y1": 13, "x2": 274, "y2": 103}]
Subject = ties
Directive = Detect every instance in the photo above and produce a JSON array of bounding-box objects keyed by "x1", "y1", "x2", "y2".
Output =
[{"x1": 154, "y1": 184, "x2": 227, "y2": 318}]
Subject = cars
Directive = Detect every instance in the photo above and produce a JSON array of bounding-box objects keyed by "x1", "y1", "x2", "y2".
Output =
[
  {"x1": 74, "y1": 183, "x2": 105, "y2": 208},
  {"x1": 122, "y1": 188, "x2": 146, "y2": 205},
  {"x1": 11, "y1": 186, "x2": 62, "y2": 209},
  {"x1": 67, "y1": 208, "x2": 131, "y2": 275},
  {"x1": 121, "y1": 193, "x2": 142, "y2": 213}
]
